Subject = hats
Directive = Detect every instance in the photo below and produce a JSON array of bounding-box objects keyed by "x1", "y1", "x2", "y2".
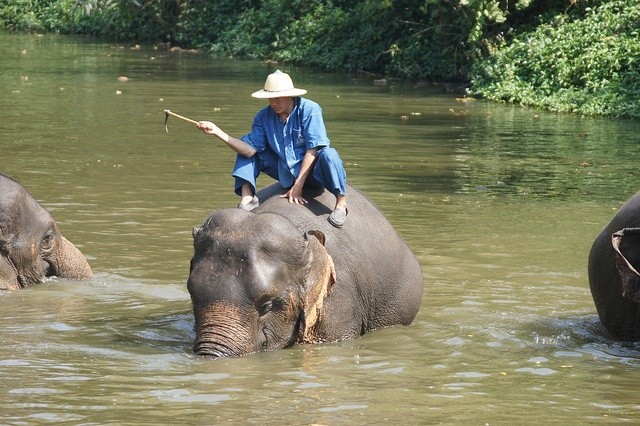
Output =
[{"x1": 251, "y1": 69, "x2": 307, "y2": 99}]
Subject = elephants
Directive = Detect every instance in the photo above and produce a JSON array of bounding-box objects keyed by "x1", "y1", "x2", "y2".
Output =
[
  {"x1": 587, "y1": 188, "x2": 640, "y2": 341},
  {"x1": 186, "y1": 176, "x2": 424, "y2": 358},
  {"x1": 1, "y1": 172, "x2": 94, "y2": 291}
]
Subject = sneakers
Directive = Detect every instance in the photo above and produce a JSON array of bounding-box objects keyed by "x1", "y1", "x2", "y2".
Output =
[
  {"x1": 238, "y1": 195, "x2": 259, "y2": 212},
  {"x1": 328, "y1": 207, "x2": 348, "y2": 227}
]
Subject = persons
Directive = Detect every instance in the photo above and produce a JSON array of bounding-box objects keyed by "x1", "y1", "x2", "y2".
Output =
[{"x1": 196, "y1": 68, "x2": 350, "y2": 228}]
